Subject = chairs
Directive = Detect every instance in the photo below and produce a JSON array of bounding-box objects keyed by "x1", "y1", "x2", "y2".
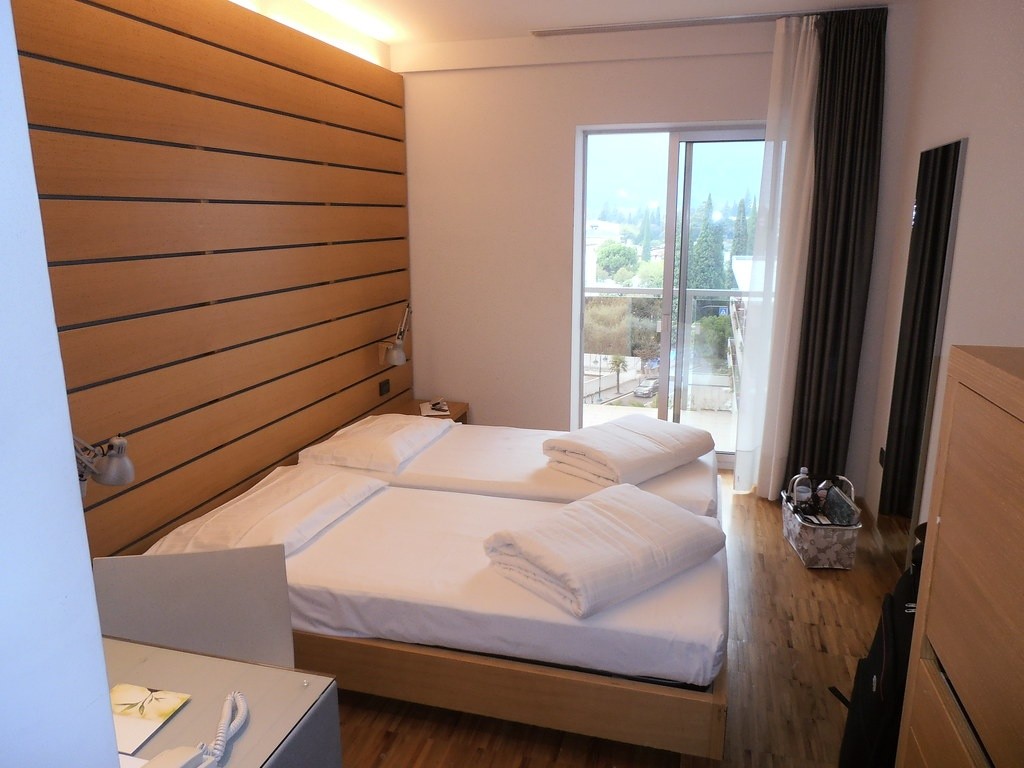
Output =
[{"x1": 93, "y1": 543, "x2": 295, "y2": 670}]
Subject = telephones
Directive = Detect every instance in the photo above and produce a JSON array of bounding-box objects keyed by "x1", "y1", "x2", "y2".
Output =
[{"x1": 141, "y1": 745, "x2": 217, "y2": 768}]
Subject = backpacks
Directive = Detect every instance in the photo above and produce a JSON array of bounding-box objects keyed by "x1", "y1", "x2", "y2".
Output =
[{"x1": 838, "y1": 522, "x2": 929, "y2": 768}]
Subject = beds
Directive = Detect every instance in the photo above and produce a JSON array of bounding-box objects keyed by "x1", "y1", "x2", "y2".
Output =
[
  {"x1": 345, "y1": 414, "x2": 718, "y2": 516},
  {"x1": 285, "y1": 485, "x2": 729, "y2": 768}
]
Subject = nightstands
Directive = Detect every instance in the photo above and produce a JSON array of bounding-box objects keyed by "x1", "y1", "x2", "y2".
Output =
[{"x1": 394, "y1": 397, "x2": 469, "y2": 423}]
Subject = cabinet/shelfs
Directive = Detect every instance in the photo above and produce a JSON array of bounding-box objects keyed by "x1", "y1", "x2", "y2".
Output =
[{"x1": 895, "y1": 344, "x2": 1024, "y2": 768}]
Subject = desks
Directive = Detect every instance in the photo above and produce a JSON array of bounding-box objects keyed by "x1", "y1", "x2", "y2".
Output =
[{"x1": 102, "y1": 633, "x2": 342, "y2": 768}]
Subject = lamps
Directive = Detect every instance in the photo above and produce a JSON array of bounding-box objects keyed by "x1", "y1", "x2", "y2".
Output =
[
  {"x1": 72, "y1": 433, "x2": 134, "y2": 498},
  {"x1": 378, "y1": 302, "x2": 413, "y2": 367}
]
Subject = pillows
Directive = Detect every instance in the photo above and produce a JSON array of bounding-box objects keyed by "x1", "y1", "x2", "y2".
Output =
[
  {"x1": 142, "y1": 463, "x2": 389, "y2": 557},
  {"x1": 299, "y1": 414, "x2": 463, "y2": 470}
]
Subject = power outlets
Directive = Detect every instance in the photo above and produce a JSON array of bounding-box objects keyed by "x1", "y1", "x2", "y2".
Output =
[{"x1": 879, "y1": 447, "x2": 885, "y2": 468}]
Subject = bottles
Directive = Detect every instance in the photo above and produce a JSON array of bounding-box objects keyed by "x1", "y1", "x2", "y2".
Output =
[
  {"x1": 794, "y1": 467, "x2": 812, "y2": 503},
  {"x1": 817, "y1": 476, "x2": 833, "y2": 506}
]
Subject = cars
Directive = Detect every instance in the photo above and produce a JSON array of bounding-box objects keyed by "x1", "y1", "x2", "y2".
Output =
[
  {"x1": 633, "y1": 376, "x2": 659, "y2": 398},
  {"x1": 641, "y1": 356, "x2": 661, "y2": 371}
]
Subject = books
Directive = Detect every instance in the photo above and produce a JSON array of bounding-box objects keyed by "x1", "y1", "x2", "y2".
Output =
[
  {"x1": 110, "y1": 682, "x2": 192, "y2": 755},
  {"x1": 419, "y1": 401, "x2": 450, "y2": 416}
]
